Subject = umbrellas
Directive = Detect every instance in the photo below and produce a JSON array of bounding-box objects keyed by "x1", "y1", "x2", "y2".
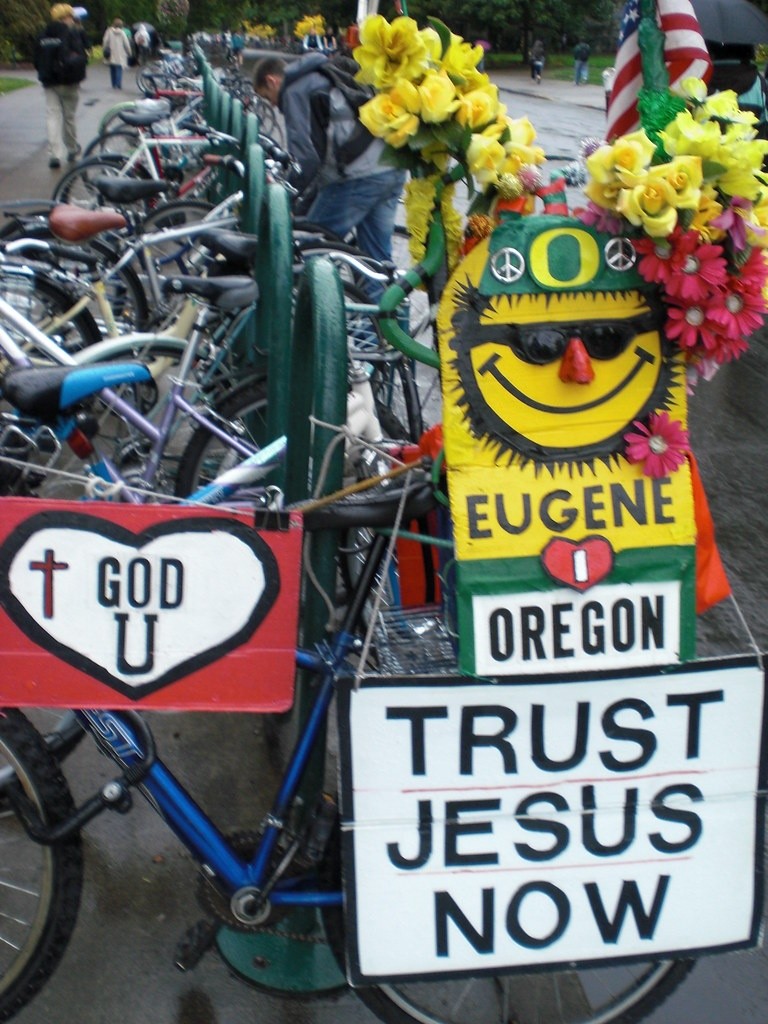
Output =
[
  {"x1": 690, "y1": 0, "x2": 768, "y2": 45},
  {"x1": 475, "y1": 39, "x2": 492, "y2": 50},
  {"x1": 132, "y1": 22, "x2": 155, "y2": 32},
  {"x1": 71, "y1": 7, "x2": 88, "y2": 17}
]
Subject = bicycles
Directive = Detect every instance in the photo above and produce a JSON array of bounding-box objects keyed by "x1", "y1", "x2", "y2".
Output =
[{"x1": 1, "y1": 34, "x2": 768, "y2": 1023}]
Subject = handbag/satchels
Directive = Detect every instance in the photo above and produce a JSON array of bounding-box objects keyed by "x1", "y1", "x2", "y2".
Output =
[
  {"x1": 103, "y1": 46, "x2": 111, "y2": 60},
  {"x1": 61, "y1": 27, "x2": 88, "y2": 68}
]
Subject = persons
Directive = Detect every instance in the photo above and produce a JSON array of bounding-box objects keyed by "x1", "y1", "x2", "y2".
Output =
[
  {"x1": 530, "y1": 39, "x2": 545, "y2": 85},
  {"x1": 252, "y1": 50, "x2": 411, "y2": 384},
  {"x1": 33, "y1": 4, "x2": 93, "y2": 170},
  {"x1": 478, "y1": 59, "x2": 485, "y2": 72},
  {"x1": 304, "y1": 24, "x2": 362, "y2": 57},
  {"x1": 101, "y1": 18, "x2": 134, "y2": 91},
  {"x1": 706, "y1": 40, "x2": 768, "y2": 173},
  {"x1": 135, "y1": 25, "x2": 164, "y2": 66},
  {"x1": 194, "y1": 29, "x2": 276, "y2": 66},
  {"x1": 573, "y1": 37, "x2": 591, "y2": 86}
]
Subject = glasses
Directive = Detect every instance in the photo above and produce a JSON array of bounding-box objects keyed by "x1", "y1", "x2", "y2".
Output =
[{"x1": 518, "y1": 322, "x2": 631, "y2": 363}]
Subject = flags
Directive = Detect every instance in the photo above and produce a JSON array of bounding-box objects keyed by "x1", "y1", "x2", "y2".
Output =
[{"x1": 606, "y1": 0, "x2": 712, "y2": 147}]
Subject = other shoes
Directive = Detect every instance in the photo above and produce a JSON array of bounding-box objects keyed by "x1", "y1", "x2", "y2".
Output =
[
  {"x1": 68, "y1": 154, "x2": 78, "y2": 163},
  {"x1": 49, "y1": 159, "x2": 60, "y2": 168}
]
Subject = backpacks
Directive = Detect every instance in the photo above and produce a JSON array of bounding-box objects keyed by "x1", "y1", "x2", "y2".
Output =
[{"x1": 279, "y1": 57, "x2": 379, "y2": 164}]
